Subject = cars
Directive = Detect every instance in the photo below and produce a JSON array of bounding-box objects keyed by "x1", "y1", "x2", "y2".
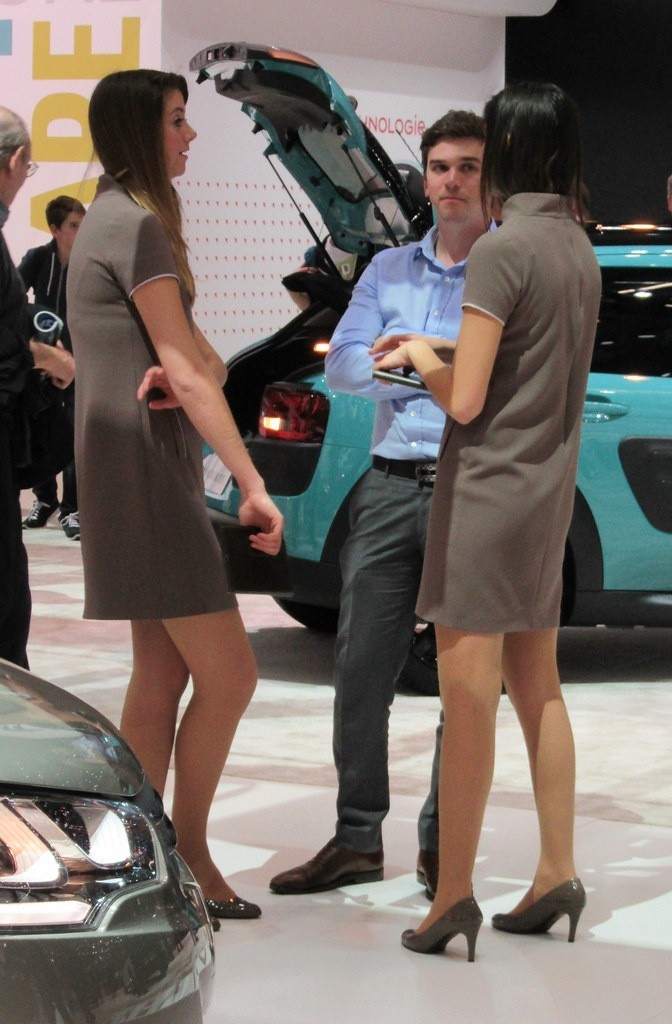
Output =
[
  {"x1": 188, "y1": 36, "x2": 672, "y2": 695},
  {"x1": 1, "y1": 659, "x2": 219, "y2": 1024}
]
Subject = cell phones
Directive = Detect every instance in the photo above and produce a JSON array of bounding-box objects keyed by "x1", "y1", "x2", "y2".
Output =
[{"x1": 220, "y1": 524, "x2": 270, "y2": 595}]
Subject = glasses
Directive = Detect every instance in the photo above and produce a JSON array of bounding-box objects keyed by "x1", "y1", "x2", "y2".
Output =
[{"x1": 24, "y1": 152, "x2": 39, "y2": 177}]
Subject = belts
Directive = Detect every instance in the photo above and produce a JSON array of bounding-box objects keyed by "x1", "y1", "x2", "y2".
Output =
[{"x1": 373, "y1": 454, "x2": 437, "y2": 484}]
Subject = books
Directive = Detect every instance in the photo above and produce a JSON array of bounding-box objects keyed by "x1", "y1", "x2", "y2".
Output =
[{"x1": 33, "y1": 309, "x2": 65, "y2": 384}]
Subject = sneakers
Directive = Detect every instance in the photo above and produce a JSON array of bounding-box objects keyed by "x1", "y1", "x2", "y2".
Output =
[
  {"x1": 22, "y1": 497, "x2": 60, "y2": 530},
  {"x1": 57, "y1": 510, "x2": 80, "y2": 541}
]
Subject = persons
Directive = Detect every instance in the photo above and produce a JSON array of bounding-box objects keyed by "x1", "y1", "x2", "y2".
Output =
[
  {"x1": 67, "y1": 70, "x2": 286, "y2": 930},
  {"x1": 15, "y1": 195, "x2": 87, "y2": 540},
  {"x1": 282, "y1": 185, "x2": 375, "y2": 312},
  {"x1": 0, "y1": 107, "x2": 75, "y2": 670},
  {"x1": 400, "y1": 84, "x2": 601, "y2": 963},
  {"x1": 268, "y1": 111, "x2": 500, "y2": 901}
]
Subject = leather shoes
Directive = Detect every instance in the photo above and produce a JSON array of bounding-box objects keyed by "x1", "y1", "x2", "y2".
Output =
[
  {"x1": 204, "y1": 893, "x2": 262, "y2": 919},
  {"x1": 269, "y1": 838, "x2": 384, "y2": 895},
  {"x1": 416, "y1": 848, "x2": 474, "y2": 903}
]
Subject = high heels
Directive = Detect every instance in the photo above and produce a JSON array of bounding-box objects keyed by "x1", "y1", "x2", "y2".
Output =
[
  {"x1": 400, "y1": 898, "x2": 484, "y2": 963},
  {"x1": 491, "y1": 876, "x2": 587, "y2": 943}
]
